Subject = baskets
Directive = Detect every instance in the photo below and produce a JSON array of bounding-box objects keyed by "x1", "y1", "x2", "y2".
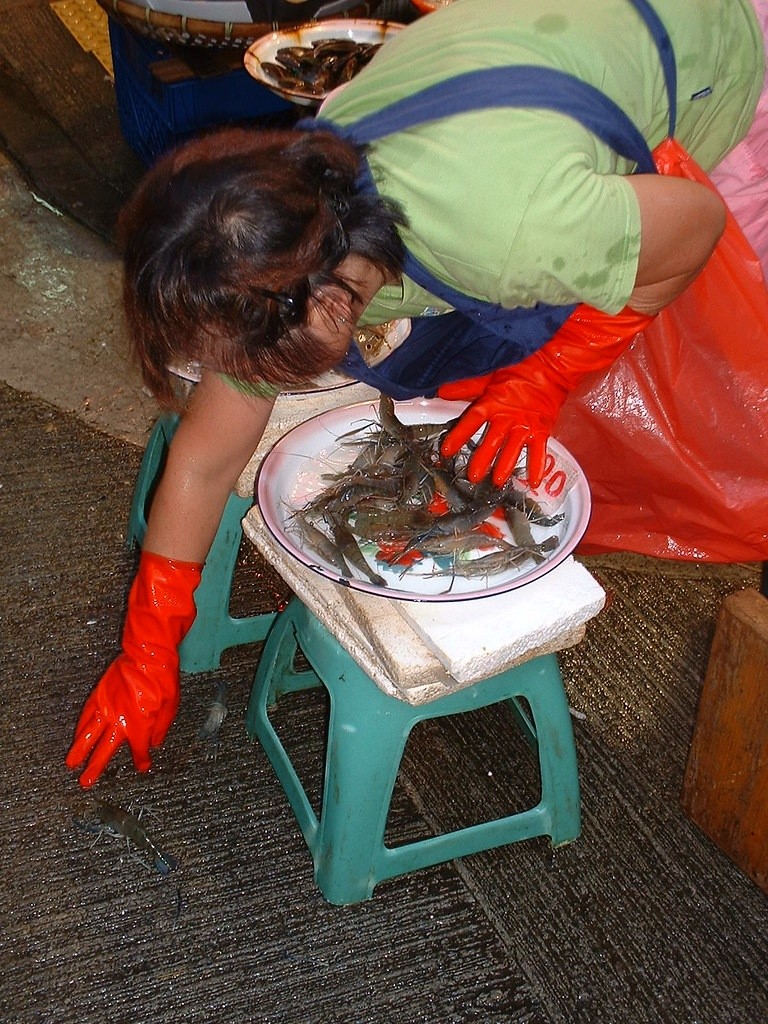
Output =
[{"x1": 97, "y1": 0, "x2": 384, "y2": 51}]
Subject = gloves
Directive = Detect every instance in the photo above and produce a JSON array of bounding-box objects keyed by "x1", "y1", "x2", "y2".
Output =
[
  {"x1": 437, "y1": 303, "x2": 659, "y2": 488},
  {"x1": 66, "y1": 554, "x2": 203, "y2": 787}
]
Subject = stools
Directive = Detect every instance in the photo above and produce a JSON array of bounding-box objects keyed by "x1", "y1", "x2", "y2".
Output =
[
  {"x1": 244, "y1": 594, "x2": 585, "y2": 906},
  {"x1": 125, "y1": 411, "x2": 277, "y2": 678}
]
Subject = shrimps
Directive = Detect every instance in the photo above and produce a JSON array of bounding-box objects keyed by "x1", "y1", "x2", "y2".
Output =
[
  {"x1": 74, "y1": 797, "x2": 179, "y2": 875},
  {"x1": 280, "y1": 387, "x2": 566, "y2": 590}
]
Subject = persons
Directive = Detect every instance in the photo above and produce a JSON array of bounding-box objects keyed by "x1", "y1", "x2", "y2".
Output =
[{"x1": 66, "y1": 0, "x2": 768, "y2": 784}]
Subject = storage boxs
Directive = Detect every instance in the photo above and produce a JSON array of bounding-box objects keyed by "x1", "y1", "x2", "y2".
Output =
[{"x1": 106, "y1": 14, "x2": 296, "y2": 168}]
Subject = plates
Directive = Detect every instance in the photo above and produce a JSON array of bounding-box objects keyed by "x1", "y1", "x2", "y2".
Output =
[
  {"x1": 243, "y1": 18, "x2": 408, "y2": 105},
  {"x1": 256, "y1": 395, "x2": 592, "y2": 602},
  {"x1": 165, "y1": 313, "x2": 413, "y2": 392}
]
